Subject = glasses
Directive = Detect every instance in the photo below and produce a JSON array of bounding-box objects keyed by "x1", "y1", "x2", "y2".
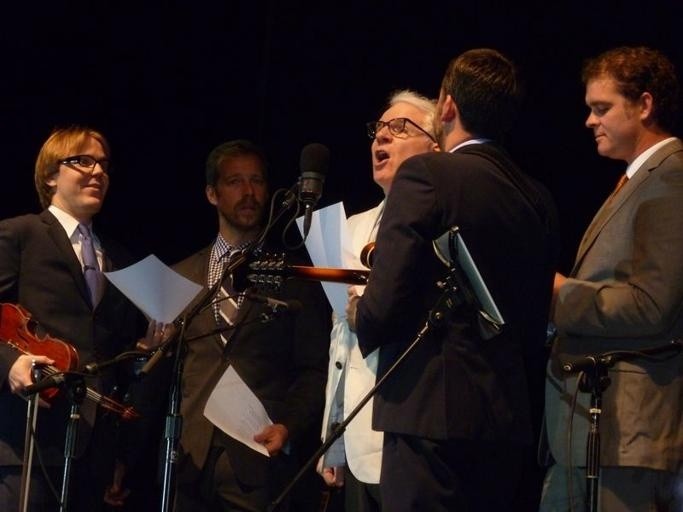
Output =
[
  {"x1": 365, "y1": 118, "x2": 435, "y2": 143},
  {"x1": 58, "y1": 155, "x2": 109, "y2": 170}
]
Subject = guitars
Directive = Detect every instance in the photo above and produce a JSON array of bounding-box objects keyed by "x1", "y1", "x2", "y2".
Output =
[{"x1": 248, "y1": 243, "x2": 376, "y2": 288}]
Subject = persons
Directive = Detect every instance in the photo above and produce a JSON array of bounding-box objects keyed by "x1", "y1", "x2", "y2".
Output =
[
  {"x1": 345, "y1": 48, "x2": 553, "y2": 511},
  {"x1": 0, "y1": 125, "x2": 177, "y2": 512},
  {"x1": 149, "y1": 140, "x2": 332, "y2": 510},
  {"x1": 317, "y1": 91, "x2": 443, "y2": 511},
  {"x1": 539, "y1": 46, "x2": 683, "y2": 512}
]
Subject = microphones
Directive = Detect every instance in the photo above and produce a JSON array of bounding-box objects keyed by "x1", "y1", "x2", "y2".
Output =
[
  {"x1": 245, "y1": 293, "x2": 288, "y2": 308},
  {"x1": 298, "y1": 143, "x2": 328, "y2": 202}
]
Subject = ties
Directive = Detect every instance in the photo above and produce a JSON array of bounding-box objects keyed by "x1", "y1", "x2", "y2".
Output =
[
  {"x1": 219, "y1": 249, "x2": 242, "y2": 341},
  {"x1": 78, "y1": 223, "x2": 101, "y2": 307},
  {"x1": 608, "y1": 173, "x2": 629, "y2": 196}
]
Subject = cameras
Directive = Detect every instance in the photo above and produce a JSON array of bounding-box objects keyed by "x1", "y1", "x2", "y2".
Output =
[{"x1": 358, "y1": 242, "x2": 479, "y2": 322}]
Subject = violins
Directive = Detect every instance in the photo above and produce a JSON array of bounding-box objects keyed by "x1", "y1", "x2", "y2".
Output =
[{"x1": 0, "y1": 301, "x2": 143, "y2": 424}]
